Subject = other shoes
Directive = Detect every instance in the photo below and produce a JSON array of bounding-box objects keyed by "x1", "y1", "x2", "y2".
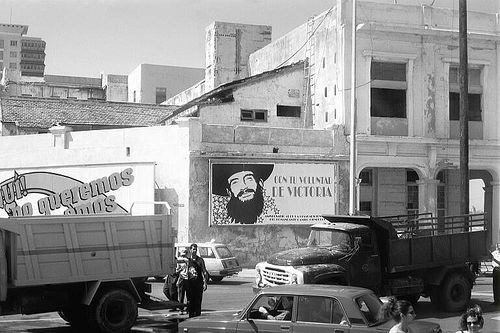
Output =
[{"x1": 189, "y1": 312, "x2": 201, "y2": 316}]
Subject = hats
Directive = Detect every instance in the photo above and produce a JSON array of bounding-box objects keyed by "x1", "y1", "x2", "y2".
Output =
[
  {"x1": 212, "y1": 164, "x2": 274, "y2": 196},
  {"x1": 181, "y1": 249, "x2": 187, "y2": 255}
]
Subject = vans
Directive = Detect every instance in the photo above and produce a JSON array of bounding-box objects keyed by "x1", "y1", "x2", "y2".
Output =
[{"x1": 153, "y1": 242, "x2": 241, "y2": 282}]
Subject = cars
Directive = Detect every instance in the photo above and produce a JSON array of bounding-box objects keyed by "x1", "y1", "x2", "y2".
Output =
[{"x1": 181, "y1": 283, "x2": 442, "y2": 333}]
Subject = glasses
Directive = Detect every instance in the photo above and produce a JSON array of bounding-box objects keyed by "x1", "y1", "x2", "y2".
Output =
[
  {"x1": 405, "y1": 311, "x2": 415, "y2": 314},
  {"x1": 466, "y1": 322, "x2": 479, "y2": 325}
]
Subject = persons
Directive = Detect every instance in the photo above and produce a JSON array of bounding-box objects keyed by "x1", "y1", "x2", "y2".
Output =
[
  {"x1": 456, "y1": 308, "x2": 484, "y2": 333},
  {"x1": 376, "y1": 298, "x2": 416, "y2": 333},
  {"x1": 489, "y1": 243, "x2": 500, "y2": 305},
  {"x1": 259, "y1": 297, "x2": 293, "y2": 321},
  {"x1": 163, "y1": 243, "x2": 209, "y2": 318}
]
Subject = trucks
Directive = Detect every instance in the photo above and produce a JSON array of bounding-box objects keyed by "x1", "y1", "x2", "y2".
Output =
[
  {"x1": 1, "y1": 211, "x2": 174, "y2": 332},
  {"x1": 256, "y1": 213, "x2": 490, "y2": 311}
]
